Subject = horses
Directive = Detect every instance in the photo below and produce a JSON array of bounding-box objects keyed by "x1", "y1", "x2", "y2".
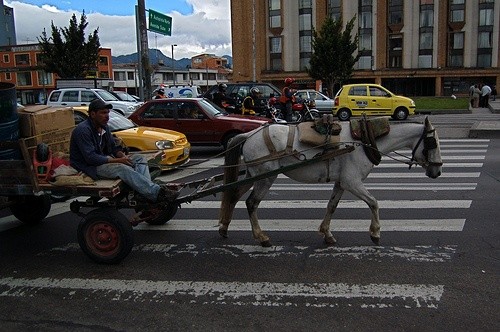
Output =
[{"x1": 219, "y1": 116, "x2": 443, "y2": 248}]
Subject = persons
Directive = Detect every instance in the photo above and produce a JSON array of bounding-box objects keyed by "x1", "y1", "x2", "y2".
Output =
[
  {"x1": 479, "y1": 84, "x2": 483, "y2": 90},
  {"x1": 234, "y1": 88, "x2": 248, "y2": 113},
  {"x1": 242, "y1": 87, "x2": 260, "y2": 115},
  {"x1": 279, "y1": 77, "x2": 298, "y2": 122},
  {"x1": 267, "y1": 97, "x2": 281, "y2": 117},
  {"x1": 480, "y1": 83, "x2": 492, "y2": 109},
  {"x1": 322, "y1": 88, "x2": 330, "y2": 99},
  {"x1": 154, "y1": 88, "x2": 168, "y2": 99},
  {"x1": 69, "y1": 98, "x2": 181, "y2": 204},
  {"x1": 213, "y1": 83, "x2": 237, "y2": 112},
  {"x1": 152, "y1": 90, "x2": 158, "y2": 99},
  {"x1": 473, "y1": 84, "x2": 480, "y2": 108},
  {"x1": 468, "y1": 82, "x2": 476, "y2": 108}
]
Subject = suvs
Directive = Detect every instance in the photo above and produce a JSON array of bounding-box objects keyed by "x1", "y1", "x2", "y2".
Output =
[
  {"x1": 197, "y1": 83, "x2": 284, "y2": 114},
  {"x1": 46, "y1": 88, "x2": 142, "y2": 119}
]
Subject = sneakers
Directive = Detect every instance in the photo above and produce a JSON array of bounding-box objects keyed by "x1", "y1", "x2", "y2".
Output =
[{"x1": 157, "y1": 186, "x2": 179, "y2": 200}]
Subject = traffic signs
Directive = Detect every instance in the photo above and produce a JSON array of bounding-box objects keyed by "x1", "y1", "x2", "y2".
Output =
[{"x1": 145, "y1": 9, "x2": 172, "y2": 36}]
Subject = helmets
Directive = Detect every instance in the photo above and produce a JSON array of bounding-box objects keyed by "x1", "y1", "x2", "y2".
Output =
[
  {"x1": 218, "y1": 83, "x2": 227, "y2": 91},
  {"x1": 284, "y1": 77, "x2": 296, "y2": 85},
  {"x1": 249, "y1": 87, "x2": 260, "y2": 95}
]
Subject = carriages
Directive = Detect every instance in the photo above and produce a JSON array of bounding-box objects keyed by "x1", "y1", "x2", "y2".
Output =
[{"x1": 0, "y1": 81, "x2": 442, "y2": 264}]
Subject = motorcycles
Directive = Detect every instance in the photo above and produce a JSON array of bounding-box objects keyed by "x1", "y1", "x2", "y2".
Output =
[
  {"x1": 271, "y1": 95, "x2": 322, "y2": 121},
  {"x1": 252, "y1": 100, "x2": 285, "y2": 122},
  {"x1": 212, "y1": 89, "x2": 242, "y2": 111}
]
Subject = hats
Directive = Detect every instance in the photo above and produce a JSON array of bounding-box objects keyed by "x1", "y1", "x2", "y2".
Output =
[{"x1": 87, "y1": 99, "x2": 112, "y2": 113}]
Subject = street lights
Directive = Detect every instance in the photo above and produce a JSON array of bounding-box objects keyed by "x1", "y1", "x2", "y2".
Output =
[{"x1": 171, "y1": 44, "x2": 176, "y2": 81}]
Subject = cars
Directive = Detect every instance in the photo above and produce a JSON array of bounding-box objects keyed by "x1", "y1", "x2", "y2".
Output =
[
  {"x1": 66, "y1": 106, "x2": 190, "y2": 176},
  {"x1": 334, "y1": 84, "x2": 415, "y2": 121},
  {"x1": 291, "y1": 90, "x2": 335, "y2": 114},
  {"x1": 111, "y1": 91, "x2": 137, "y2": 102},
  {"x1": 127, "y1": 98, "x2": 272, "y2": 152}
]
